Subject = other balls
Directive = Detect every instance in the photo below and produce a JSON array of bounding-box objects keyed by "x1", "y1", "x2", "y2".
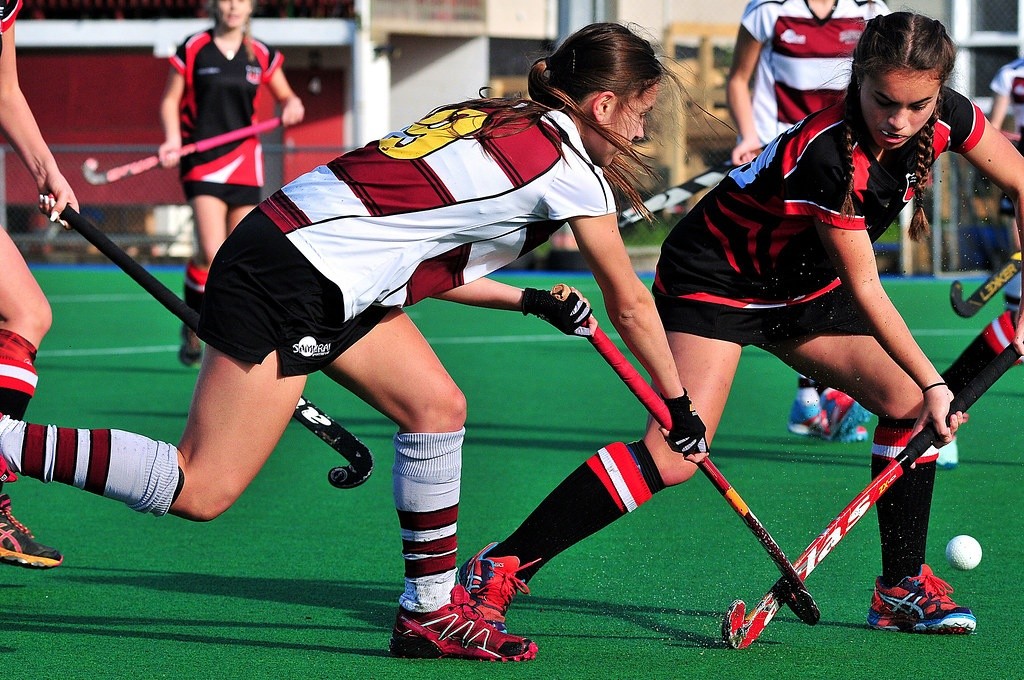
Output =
[{"x1": 945, "y1": 535, "x2": 982, "y2": 570}]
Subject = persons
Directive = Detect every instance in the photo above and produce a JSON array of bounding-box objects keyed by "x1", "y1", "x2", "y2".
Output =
[
  {"x1": 937, "y1": 311, "x2": 1024, "y2": 467},
  {"x1": 159, "y1": 0, "x2": 306, "y2": 366},
  {"x1": 988, "y1": 59, "x2": 1024, "y2": 315},
  {"x1": 452, "y1": 13, "x2": 1024, "y2": 634},
  {"x1": 0, "y1": 23, "x2": 706, "y2": 660},
  {"x1": 726, "y1": 0, "x2": 889, "y2": 444},
  {"x1": 0, "y1": 0, "x2": 79, "y2": 568}
]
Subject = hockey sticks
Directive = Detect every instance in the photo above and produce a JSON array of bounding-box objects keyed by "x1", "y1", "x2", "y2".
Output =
[
  {"x1": 721, "y1": 340, "x2": 1023, "y2": 650},
  {"x1": 950, "y1": 251, "x2": 1022, "y2": 319},
  {"x1": 615, "y1": 145, "x2": 767, "y2": 233},
  {"x1": 549, "y1": 282, "x2": 822, "y2": 629},
  {"x1": 40, "y1": 189, "x2": 376, "y2": 490},
  {"x1": 82, "y1": 118, "x2": 284, "y2": 186}
]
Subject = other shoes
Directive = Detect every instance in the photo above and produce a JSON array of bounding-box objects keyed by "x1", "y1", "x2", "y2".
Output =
[
  {"x1": 936, "y1": 436, "x2": 959, "y2": 468},
  {"x1": 180, "y1": 324, "x2": 201, "y2": 364}
]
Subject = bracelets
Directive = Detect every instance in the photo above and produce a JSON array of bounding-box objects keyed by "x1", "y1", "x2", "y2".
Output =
[{"x1": 922, "y1": 383, "x2": 948, "y2": 393}]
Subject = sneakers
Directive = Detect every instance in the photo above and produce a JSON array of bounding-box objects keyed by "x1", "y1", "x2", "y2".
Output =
[
  {"x1": 867, "y1": 564, "x2": 976, "y2": 634},
  {"x1": 457, "y1": 542, "x2": 542, "y2": 634},
  {"x1": 819, "y1": 386, "x2": 874, "y2": 441},
  {"x1": 0, "y1": 455, "x2": 64, "y2": 567},
  {"x1": 389, "y1": 584, "x2": 539, "y2": 662},
  {"x1": 788, "y1": 377, "x2": 866, "y2": 444}
]
